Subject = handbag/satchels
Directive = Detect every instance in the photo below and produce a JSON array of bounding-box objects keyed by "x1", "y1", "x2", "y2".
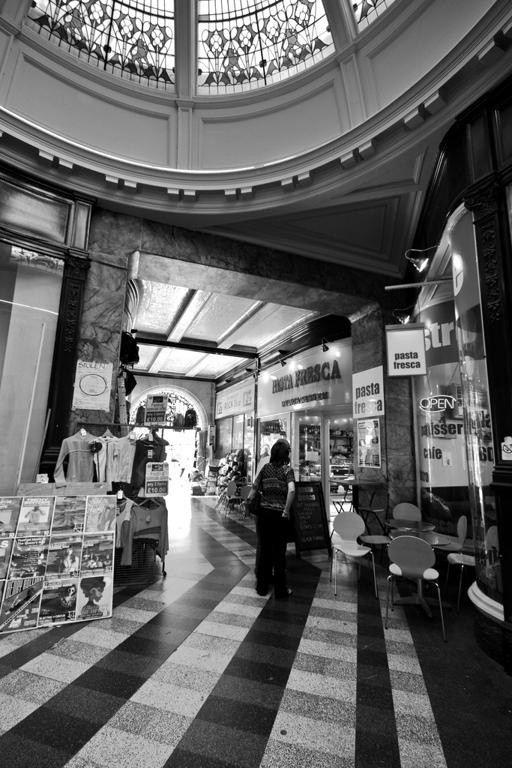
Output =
[{"x1": 243, "y1": 493, "x2": 261, "y2": 513}]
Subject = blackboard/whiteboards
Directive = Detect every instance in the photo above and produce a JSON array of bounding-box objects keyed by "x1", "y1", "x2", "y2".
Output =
[{"x1": 289, "y1": 481, "x2": 330, "y2": 551}]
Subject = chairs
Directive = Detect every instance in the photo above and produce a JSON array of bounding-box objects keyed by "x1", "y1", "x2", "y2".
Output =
[
  {"x1": 330, "y1": 488, "x2": 353, "y2": 539},
  {"x1": 218, "y1": 477, "x2": 252, "y2": 520},
  {"x1": 330, "y1": 503, "x2": 500, "y2": 643}
]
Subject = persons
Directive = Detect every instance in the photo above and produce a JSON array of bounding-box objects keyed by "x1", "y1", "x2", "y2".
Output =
[
  {"x1": 25, "y1": 503, "x2": 46, "y2": 522},
  {"x1": 244, "y1": 439, "x2": 296, "y2": 601},
  {"x1": 53, "y1": 584, "x2": 77, "y2": 612}
]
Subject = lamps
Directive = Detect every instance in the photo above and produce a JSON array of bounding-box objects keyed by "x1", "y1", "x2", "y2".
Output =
[
  {"x1": 405, "y1": 245, "x2": 439, "y2": 272},
  {"x1": 278, "y1": 354, "x2": 287, "y2": 367},
  {"x1": 320, "y1": 337, "x2": 330, "y2": 352}
]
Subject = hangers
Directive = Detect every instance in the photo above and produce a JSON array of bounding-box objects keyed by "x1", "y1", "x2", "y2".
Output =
[
  {"x1": 131, "y1": 497, "x2": 167, "y2": 515},
  {"x1": 109, "y1": 486, "x2": 135, "y2": 510},
  {"x1": 71, "y1": 421, "x2": 167, "y2": 451}
]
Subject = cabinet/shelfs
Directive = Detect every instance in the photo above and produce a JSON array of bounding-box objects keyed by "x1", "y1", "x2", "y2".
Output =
[{"x1": 299, "y1": 435, "x2": 353, "y2": 456}]
